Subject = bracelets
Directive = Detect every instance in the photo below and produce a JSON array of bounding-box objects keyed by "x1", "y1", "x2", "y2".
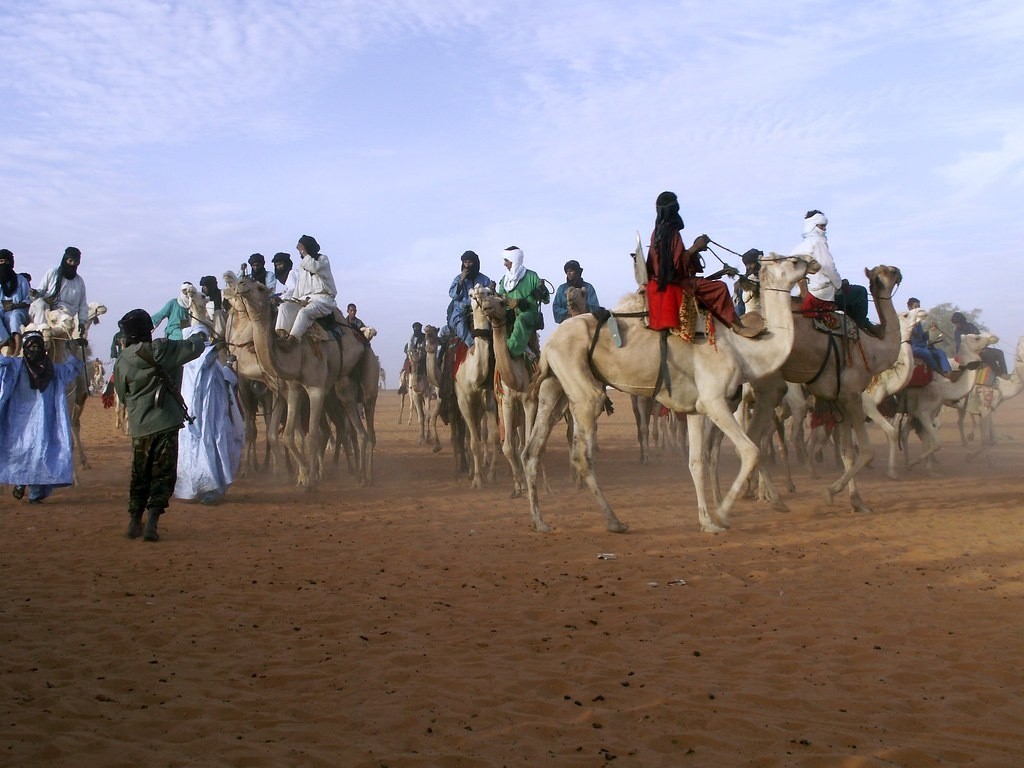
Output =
[
  {"x1": 301, "y1": 250, "x2": 305, "y2": 253},
  {"x1": 226, "y1": 361, "x2": 233, "y2": 365}
]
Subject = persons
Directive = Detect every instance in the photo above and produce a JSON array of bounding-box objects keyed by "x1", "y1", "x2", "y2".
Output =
[
  {"x1": 438, "y1": 245, "x2": 550, "y2": 366},
  {"x1": 731, "y1": 248, "x2": 763, "y2": 314},
  {"x1": 171, "y1": 325, "x2": 244, "y2": 506},
  {"x1": 645, "y1": 191, "x2": 765, "y2": 337},
  {"x1": 792, "y1": 210, "x2": 885, "y2": 338},
  {"x1": 551, "y1": 260, "x2": 599, "y2": 324},
  {"x1": 0, "y1": 246, "x2": 104, "y2": 507},
  {"x1": 114, "y1": 310, "x2": 207, "y2": 541},
  {"x1": 110, "y1": 320, "x2": 126, "y2": 358},
  {"x1": 907, "y1": 298, "x2": 951, "y2": 377},
  {"x1": 151, "y1": 235, "x2": 337, "y2": 360},
  {"x1": 950, "y1": 312, "x2": 1007, "y2": 376},
  {"x1": 345, "y1": 303, "x2": 365, "y2": 328},
  {"x1": 403, "y1": 322, "x2": 426, "y2": 373}
]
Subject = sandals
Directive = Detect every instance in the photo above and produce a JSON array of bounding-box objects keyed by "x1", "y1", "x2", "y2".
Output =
[
  {"x1": 12, "y1": 485, "x2": 24, "y2": 500},
  {"x1": 29, "y1": 498, "x2": 40, "y2": 505}
]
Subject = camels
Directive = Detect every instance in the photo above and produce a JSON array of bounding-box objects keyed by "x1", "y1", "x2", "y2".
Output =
[
  {"x1": 186, "y1": 271, "x2": 385, "y2": 491},
  {"x1": 1, "y1": 297, "x2": 108, "y2": 487},
  {"x1": 397, "y1": 249, "x2": 1024, "y2": 533}
]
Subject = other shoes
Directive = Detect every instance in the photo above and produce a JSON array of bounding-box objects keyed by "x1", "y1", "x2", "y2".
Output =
[
  {"x1": 127, "y1": 522, "x2": 143, "y2": 540},
  {"x1": 142, "y1": 526, "x2": 160, "y2": 542}
]
